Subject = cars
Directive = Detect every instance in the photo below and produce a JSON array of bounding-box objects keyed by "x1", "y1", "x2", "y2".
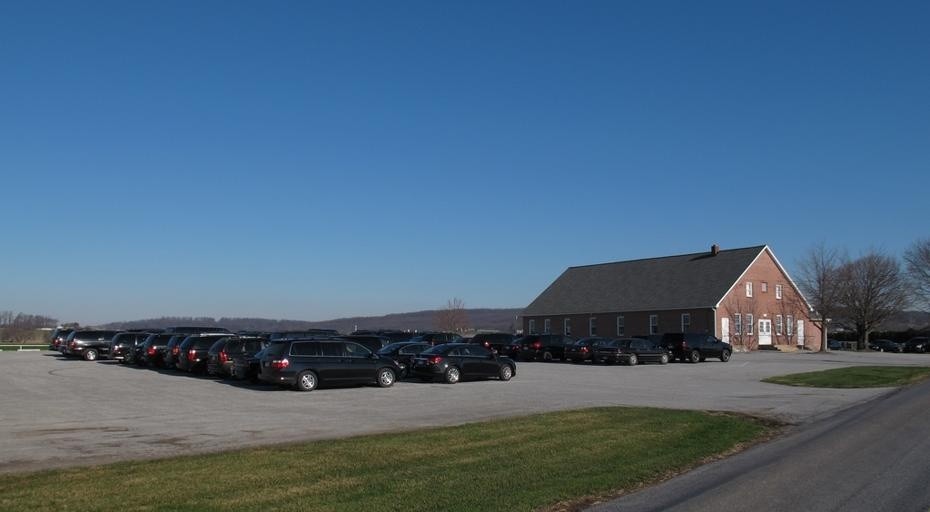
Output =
[{"x1": 827, "y1": 335, "x2": 930, "y2": 355}]
